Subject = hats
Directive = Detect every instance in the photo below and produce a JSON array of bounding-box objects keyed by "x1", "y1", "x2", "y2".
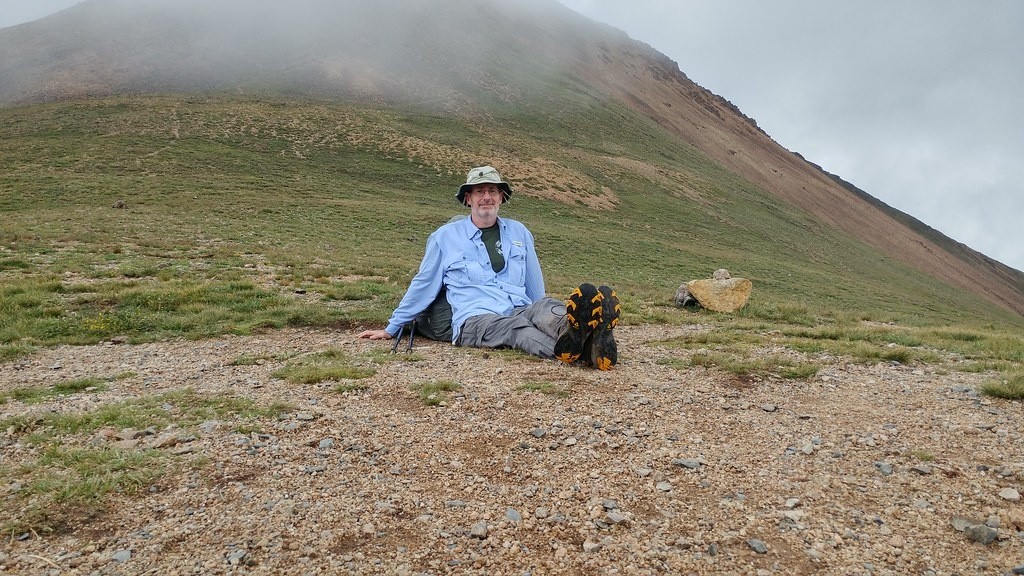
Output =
[{"x1": 454, "y1": 166, "x2": 514, "y2": 207}]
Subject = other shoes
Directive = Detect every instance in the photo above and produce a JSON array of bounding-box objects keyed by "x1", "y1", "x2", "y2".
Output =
[
  {"x1": 554, "y1": 283, "x2": 603, "y2": 363},
  {"x1": 589, "y1": 286, "x2": 622, "y2": 371}
]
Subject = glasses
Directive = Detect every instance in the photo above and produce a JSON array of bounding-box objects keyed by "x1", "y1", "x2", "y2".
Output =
[{"x1": 471, "y1": 189, "x2": 500, "y2": 197}]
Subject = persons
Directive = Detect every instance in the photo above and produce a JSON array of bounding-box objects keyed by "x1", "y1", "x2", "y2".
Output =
[{"x1": 358, "y1": 166, "x2": 621, "y2": 371}]
